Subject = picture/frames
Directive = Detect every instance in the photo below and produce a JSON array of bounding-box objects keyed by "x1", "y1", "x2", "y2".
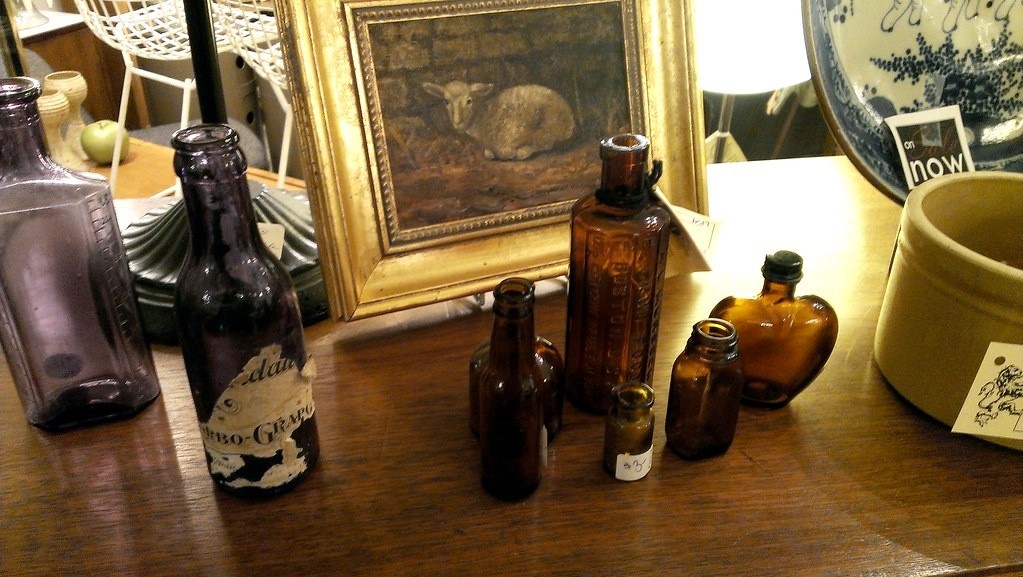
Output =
[{"x1": 268, "y1": 0, "x2": 712, "y2": 328}]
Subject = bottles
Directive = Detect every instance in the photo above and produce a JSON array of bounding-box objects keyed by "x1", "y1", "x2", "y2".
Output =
[
  {"x1": 601, "y1": 381, "x2": 655, "y2": 482},
  {"x1": 708, "y1": 250, "x2": 838, "y2": 410},
  {"x1": 1, "y1": 77, "x2": 160, "y2": 436},
  {"x1": 468, "y1": 337, "x2": 563, "y2": 440},
  {"x1": 170, "y1": 123, "x2": 319, "y2": 500},
  {"x1": 475, "y1": 278, "x2": 547, "y2": 503},
  {"x1": 560, "y1": 134, "x2": 671, "y2": 414},
  {"x1": 664, "y1": 317, "x2": 744, "y2": 460}
]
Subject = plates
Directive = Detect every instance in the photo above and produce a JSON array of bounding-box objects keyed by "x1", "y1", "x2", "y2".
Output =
[{"x1": 800, "y1": 1, "x2": 1023, "y2": 206}]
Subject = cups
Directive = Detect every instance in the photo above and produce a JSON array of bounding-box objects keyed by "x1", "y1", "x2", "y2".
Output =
[
  {"x1": 875, "y1": 172, "x2": 1023, "y2": 450},
  {"x1": 43, "y1": 70, "x2": 93, "y2": 160},
  {"x1": 37, "y1": 88, "x2": 89, "y2": 172}
]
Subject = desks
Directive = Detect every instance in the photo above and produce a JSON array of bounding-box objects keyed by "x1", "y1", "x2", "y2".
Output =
[{"x1": 2, "y1": 124, "x2": 1022, "y2": 574}]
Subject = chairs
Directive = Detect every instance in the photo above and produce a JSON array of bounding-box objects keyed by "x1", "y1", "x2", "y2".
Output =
[{"x1": 71, "y1": 0, "x2": 295, "y2": 199}]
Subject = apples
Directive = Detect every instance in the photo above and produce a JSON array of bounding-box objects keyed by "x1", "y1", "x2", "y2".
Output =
[{"x1": 80, "y1": 119, "x2": 129, "y2": 164}]
